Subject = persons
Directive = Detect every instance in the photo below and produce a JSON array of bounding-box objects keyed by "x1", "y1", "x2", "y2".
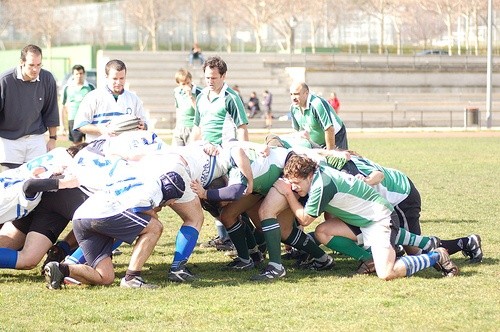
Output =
[
  {"x1": 171, "y1": 66, "x2": 204, "y2": 146},
  {"x1": 289, "y1": 82, "x2": 348, "y2": 151},
  {"x1": 0, "y1": 40, "x2": 482, "y2": 289},
  {"x1": 233, "y1": 84, "x2": 244, "y2": 105},
  {"x1": 187, "y1": 56, "x2": 250, "y2": 144},
  {"x1": 0, "y1": 44, "x2": 59, "y2": 174},
  {"x1": 263, "y1": 89, "x2": 273, "y2": 128},
  {"x1": 61, "y1": 64, "x2": 97, "y2": 147},
  {"x1": 73, "y1": 59, "x2": 153, "y2": 144},
  {"x1": 188, "y1": 43, "x2": 206, "y2": 66},
  {"x1": 247, "y1": 91, "x2": 260, "y2": 119}
]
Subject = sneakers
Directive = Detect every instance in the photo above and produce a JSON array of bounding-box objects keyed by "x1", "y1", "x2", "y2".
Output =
[
  {"x1": 357, "y1": 261, "x2": 375, "y2": 274},
  {"x1": 221, "y1": 257, "x2": 254, "y2": 270},
  {"x1": 283, "y1": 247, "x2": 308, "y2": 259},
  {"x1": 200, "y1": 236, "x2": 222, "y2": 246},
  {"x1": 169, "y1": 260, "x2": 204, "y2": 281},
  {"x1": 42, "y1": 246, "x2": 64, "y2": 277},
  {"x1": 59, "y1": 255, "x2": 83, "y2": 285},
  {"x1": 120, "y1": 276, "x2": 159, "y2": 289},
  {"x1": 249, "y1": 262, "x2": 286, "y2": 280},
  {"x1": 217, "y1": 240, "x2": 232, "y2": 250},
  {"x1": 432, "y1": 247, "x2": 458, "y2": 278},
  {"x1": 396, "y1": 245, "x2": 407, "y2": 257},
  {"x1": 233, "y1": 252, "x2": 264, "y2": 263},
  {"x1": 462, "y1": 234, "x2": 483, "y2": 264},
  {"x1": 43, "y1": 261, "x2": 63, "y2": 291},
  {"x1": 259, "y1": 242, "x2": 268, "y2": 255},
  {"x1": 291, "y1": 252, "x2": 336, "y2": 270},
  {"x1": 422, "y1": 236, "x2": 441, "y2": 254}
]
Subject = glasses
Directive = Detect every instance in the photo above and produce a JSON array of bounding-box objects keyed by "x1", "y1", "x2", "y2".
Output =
[{"x1": 26, "y1": 63, "x2": 44, "y2": 69}]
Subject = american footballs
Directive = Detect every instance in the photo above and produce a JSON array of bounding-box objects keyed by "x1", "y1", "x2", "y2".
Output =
[{"x1": 107, "y1": 115, "x2": 141, "y2": 136}]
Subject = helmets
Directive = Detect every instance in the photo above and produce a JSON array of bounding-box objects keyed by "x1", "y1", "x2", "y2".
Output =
[{"x1": 161, "y1": 171, "x2": 185, "y2": 200}]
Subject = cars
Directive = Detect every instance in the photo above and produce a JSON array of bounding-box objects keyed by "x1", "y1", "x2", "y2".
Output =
[{"x1": 416, "y1": 49, "x2": 448, "y2": 56}]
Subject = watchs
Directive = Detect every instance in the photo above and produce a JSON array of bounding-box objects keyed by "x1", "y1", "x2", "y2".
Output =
[{"x1": 48, "y1": 134, "x2": 56, "y2": 140}]
